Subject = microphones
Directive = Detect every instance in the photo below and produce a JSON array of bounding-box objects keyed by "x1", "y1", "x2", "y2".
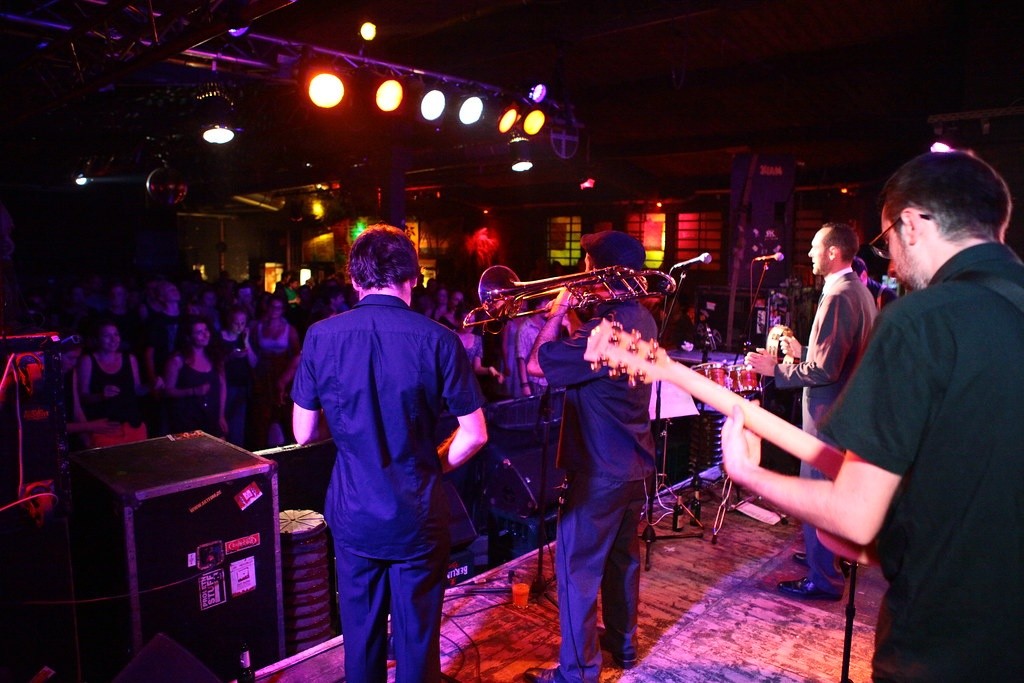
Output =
[
  {"x1": 754, "y1": 253, "x2": 784, "y2": 262},
  {"x1": 673, "y1": 252, "x2": 712, "y2": 269}
]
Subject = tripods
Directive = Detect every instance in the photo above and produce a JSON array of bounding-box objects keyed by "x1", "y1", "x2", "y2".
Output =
[
  {"x1": 699, "y1": 471, "x2": 790, "y2": 545},
  {"x1": 635, "y1": 268, "x2": 703, "y2": 571},
  {"x1": 464, "y1": 383, "x2": 561, "y2": 611}
]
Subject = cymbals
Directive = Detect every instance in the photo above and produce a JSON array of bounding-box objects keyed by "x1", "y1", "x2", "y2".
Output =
[{"x1": 766, "y1": 324, "x2": 794, "y2": 365}]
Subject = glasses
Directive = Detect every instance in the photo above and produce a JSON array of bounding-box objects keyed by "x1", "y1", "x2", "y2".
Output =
[
  {"x1": 56, "y1": 334, "x2": 81, "y2": 347},
  {"x1": 868, "y1": 213, "x2": 933, "y2": 260}
]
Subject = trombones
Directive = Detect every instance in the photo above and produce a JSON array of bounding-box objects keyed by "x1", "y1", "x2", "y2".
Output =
[{"x1": 463, "y1": 266, "x2": 676, "y2": 335}]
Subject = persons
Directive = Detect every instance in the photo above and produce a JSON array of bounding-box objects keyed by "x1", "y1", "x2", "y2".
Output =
[
  {"x1": 473, "y1": 263, "x2": 552, "y2": 399},
  {"x1": 524, "y1": 230, "x2": 658, "y2": 683},
  {"x1": 293, "y1": 227, "x2": 486, "y2": 683},
  {"x1": 721, "y1": 149, "x2": 1024, "y2": 683},
  {"x1": 0, "y1": 200, "x2": 697, "y2": 449},
  {"x1": 746, "y1": 220, "x2": 900, "y2": 599}
]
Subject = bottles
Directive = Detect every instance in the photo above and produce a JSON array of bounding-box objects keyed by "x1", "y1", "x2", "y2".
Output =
[
  {"x1": 690, "y1": 487, "x2": 700, "y2": 526},
  {"x1": 672, "y1": 491, "x2": 685, "y2": 533},
  {"x1": 237, "y1": 642, "x2": 255, "y2": 683}
]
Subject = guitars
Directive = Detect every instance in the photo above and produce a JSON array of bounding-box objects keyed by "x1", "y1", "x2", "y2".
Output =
[
  {"x1": 584, "y1": 318, "x2": 878, "y2": 567},
  {"x1": 444, "y1": 478, "x2": 477, "y2": 556}
]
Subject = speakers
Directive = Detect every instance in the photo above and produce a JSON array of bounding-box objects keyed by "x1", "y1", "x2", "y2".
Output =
[
  {"x1": 110, "y1": 632, "x2": 219, "y2": 683},
  {"x1": 485, "y1": 386, "x2": 569, "y2": 516},
  {"x1": 487, "y1": 503, "x2": 559, "y2": 568}
]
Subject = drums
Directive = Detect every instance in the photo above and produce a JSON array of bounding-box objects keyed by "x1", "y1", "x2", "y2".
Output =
[
  {"x1": 725, "y1": 364, "x2": 760, "y2": 397},
  {"x1": 691, "y1": 362, "x2": 729, "y2": 403}
]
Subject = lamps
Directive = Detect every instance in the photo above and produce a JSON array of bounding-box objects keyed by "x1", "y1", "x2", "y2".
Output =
[{"x1": 144, "y1": 59, "x2": 547, "y2": 207}]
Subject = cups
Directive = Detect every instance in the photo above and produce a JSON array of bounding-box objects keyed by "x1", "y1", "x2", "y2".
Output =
[{"x1": 511, "y1": 578, "x2": 531, "y2": 611}]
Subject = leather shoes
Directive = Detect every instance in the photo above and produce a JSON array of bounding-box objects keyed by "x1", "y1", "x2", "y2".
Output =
[
  {"x1": 792, "y1": 553, "x2": 851, "y2": 578},
  {"x1": 777, "y1": 574, "x2": 843, "y2": 601},
  {"x1": 522, "y1": 667, "x2": 555, "y2": 683},
  {"x1": 595, "y1": 626, "x2": 637, "y2": 667}
]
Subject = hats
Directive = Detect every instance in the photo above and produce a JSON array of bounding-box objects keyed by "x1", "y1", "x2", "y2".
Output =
[{"x1": 580, "y1": 230, "x2": 646, "y2": 272}]
